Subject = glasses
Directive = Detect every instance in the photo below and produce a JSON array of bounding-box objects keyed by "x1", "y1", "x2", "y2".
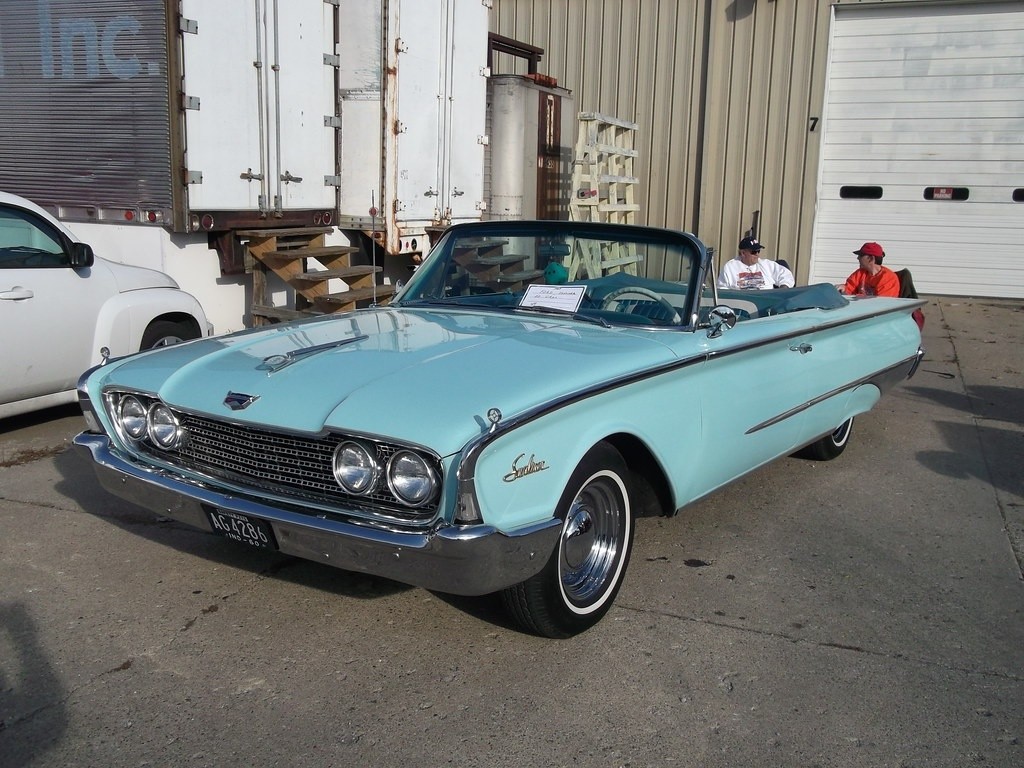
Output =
[{"x1": 751, "y1": 250, "x2": 761, "y2": 255}]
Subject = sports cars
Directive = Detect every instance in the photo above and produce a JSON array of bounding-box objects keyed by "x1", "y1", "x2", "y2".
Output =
[{"x1": 72, "y1": 218, "x2": 931, "y2": 639}]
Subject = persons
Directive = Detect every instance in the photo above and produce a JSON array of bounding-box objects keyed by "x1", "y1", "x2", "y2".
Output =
[
  {"x1": 717, "y1": 236, "x2": 796, "y2": 290},
  {"x1": 835, "y1": 241, "x2": 901, "y2": 298}
]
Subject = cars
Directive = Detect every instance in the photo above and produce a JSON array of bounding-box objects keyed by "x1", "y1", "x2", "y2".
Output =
[{"x1": 0, "y1": 190, "x2": 213, "y2": 421}]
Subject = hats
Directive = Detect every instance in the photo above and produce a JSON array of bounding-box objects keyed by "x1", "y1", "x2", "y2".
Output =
[
  {"x1": 738, "y1": 236, "x2": 765, "y2": 252},
  {"x1": 852, "y1": 242, "x2": 883, "y2": 258}
]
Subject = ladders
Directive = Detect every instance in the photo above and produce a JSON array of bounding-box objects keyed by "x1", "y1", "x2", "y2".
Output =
[{"x1": 563, "y1": 111, "x2": 645, "y2": 283}]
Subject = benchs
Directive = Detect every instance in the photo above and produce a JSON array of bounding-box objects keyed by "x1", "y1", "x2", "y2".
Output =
[{"x1": 589, "y1": 286, "x2": 759, "y2": 327}]
